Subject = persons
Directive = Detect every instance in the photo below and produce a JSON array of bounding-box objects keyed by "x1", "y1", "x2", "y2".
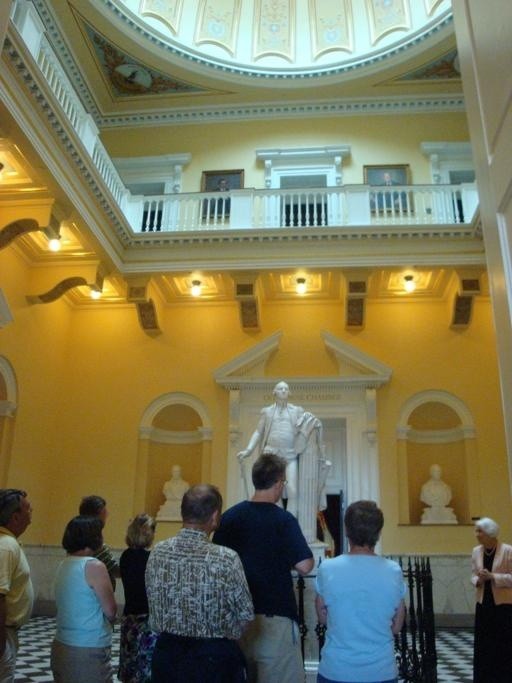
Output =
[
  {"x1": 116, "y1": 511, "x2": 157, "y2": 681},
  {"x1": 208, "y1": 451, "x2": 316, "y2": 682},
  {"x1": 370, "y1": 169, "x2": 408, "y2": 209},
  {"x1": 143, "y1": 481, "x2": 256, "y2": 682},
  {"x1": 210, "y1": 177, "x2": 231, "y2": 214},
  {"x1": 235, "y1": 380, "x2": 325, "y2": 542},
  {"x1": 50, "y1": 514, "x2": 120, "y2": 682},
  {"x1": 161, "y1": 463, "x2": 191, "y2": 504},
  {"x1": 418, "y1": 463, "x2": 452, "y2": 509},
  {"x1": 315, "y1": 498, "x2": 407, "y2": 683},
  {"x1": 1, "y1": 486, "x2": 35, "y2": 682},
  {"x1": 76, "y1": 494, "x2": 123, "y2": 595},
  {"x1": 469, "y1": 516, "x2": 512, "y2": 682}
]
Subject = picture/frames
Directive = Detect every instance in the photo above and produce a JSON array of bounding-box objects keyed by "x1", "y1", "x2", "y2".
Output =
[
  {"x1": 362, "y1": 163, "x2": 413, "y2": 212},
  {"x1": 199, "y1": 168, "x2": 245, "y2": 219}
]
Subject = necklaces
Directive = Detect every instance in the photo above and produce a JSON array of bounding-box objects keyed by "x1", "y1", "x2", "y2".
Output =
[{"x1": 484, "y1": 544, "x2": 496, "y2": 556}]
